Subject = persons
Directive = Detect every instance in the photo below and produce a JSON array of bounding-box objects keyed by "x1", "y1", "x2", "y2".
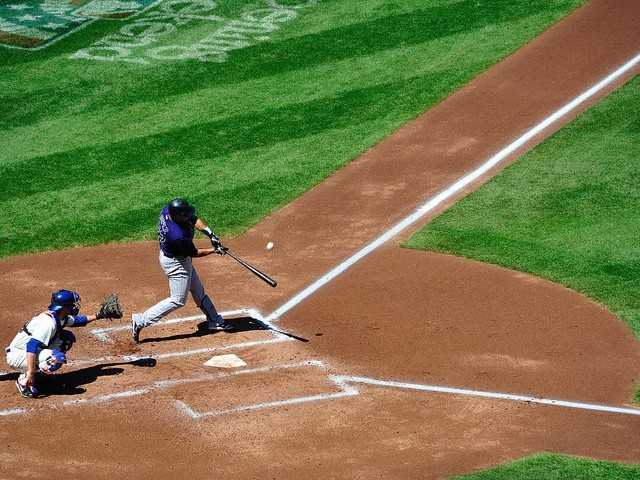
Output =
[
  {"x1": 5, "y1": 290, "x2": 123, "y2": 398},
  {"x1": 131, "y1": 198, "x2": 234, "y2": 343}
]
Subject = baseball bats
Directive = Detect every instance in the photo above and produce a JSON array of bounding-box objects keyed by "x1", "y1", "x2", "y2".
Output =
[{"x1": 217, "y1": 245, "x2": 277, "y2": 287}]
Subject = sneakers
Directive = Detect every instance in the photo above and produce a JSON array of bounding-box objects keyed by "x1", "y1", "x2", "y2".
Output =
[
  {"x1": 131, "y1": 314, "x2": 144, "y2": 343},
  {"x1": 15, "y1": 374, "x2": 35, "y2": 398},
  {"x1": 208, "y1": 321, "x2": 235, "y2": 331}
]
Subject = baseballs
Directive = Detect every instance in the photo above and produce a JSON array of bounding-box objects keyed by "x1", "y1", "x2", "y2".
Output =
[{"x1": 266, "y1": 242, "x2": 274, "y2": 250}]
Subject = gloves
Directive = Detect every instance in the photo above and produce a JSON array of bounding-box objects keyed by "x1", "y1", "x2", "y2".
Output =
[
  {"x1": 209, "y1": 233, "x2": 221, "y2": 248},
  {"x1": 215, "y1": 244, "x2": 228, "y2": 255}
]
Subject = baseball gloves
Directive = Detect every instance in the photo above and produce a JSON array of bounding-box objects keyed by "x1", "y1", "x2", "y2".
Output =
[{"x1": 95, "y1": 292, "x2": 123, "y2": 322}]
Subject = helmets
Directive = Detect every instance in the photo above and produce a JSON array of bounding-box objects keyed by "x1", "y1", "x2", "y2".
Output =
[
  {"x1": 48, "y1": 289, "x2": 82, "y2": 316},
  {"x1": 168, "y1": 198, "x2": 195, "y2": 225}
]
